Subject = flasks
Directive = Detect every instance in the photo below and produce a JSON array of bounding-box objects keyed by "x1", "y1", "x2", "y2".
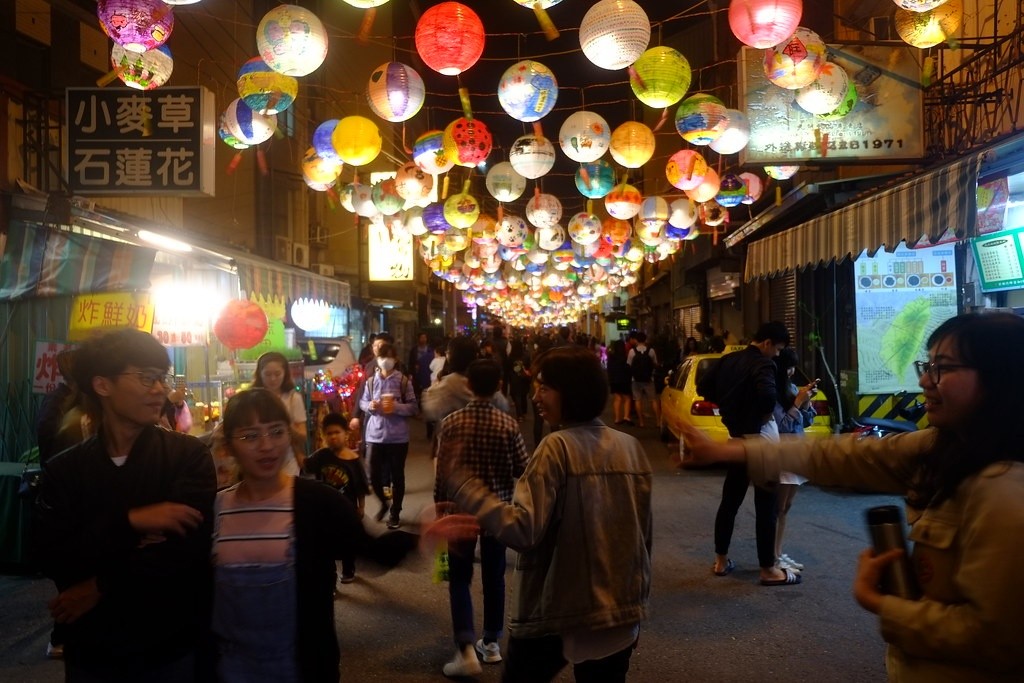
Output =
[{"x1": 863, "y1": 504, "x2": 912, "y2": 601}]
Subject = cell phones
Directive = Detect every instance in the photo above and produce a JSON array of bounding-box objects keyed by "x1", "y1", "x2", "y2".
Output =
[{"x1": 811, "y1": 379, "x2": 821, "y2": 387}]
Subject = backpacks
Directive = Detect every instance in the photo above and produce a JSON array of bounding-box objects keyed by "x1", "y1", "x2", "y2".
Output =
[{"x1": 631, "y1": 346, "x2": 653, "y2": 383}]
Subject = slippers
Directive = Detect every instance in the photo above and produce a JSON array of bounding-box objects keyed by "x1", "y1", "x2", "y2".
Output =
[
  {"x1": 759, "y1": 568, "x2": 802, "y2": 585},
  {"x1": 713, "y1": 558, "x2": 731, "y2": 576}
]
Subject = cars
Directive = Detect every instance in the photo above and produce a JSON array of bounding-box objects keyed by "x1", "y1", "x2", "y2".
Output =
[{"x1": 658, "y1": 345, "x2": 833, "y2": 473}]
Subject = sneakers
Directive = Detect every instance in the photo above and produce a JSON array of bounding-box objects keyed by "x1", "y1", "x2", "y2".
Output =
[
  {"x1": 775, "y1": 557, "x2": 800, "y2": 576},
  {"x1": 442, "y1": 647, "x2": 482, "y2": 678},
  {"x1": 477, "y1": 639, "x2": 502, "y2": 662},
  {"x1": 781, "y1": 553, "x2": 804, "y2": 570}
]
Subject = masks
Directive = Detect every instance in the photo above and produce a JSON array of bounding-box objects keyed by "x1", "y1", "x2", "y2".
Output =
[{"x1": 377, "y1": 358, "x2": 395, "y2": 371}]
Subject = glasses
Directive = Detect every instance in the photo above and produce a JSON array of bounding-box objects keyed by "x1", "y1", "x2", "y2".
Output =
[
  {"x1": 118, "y1": 370, "x2": 176, "y2": 390},
  {"x1": 913, "y1": 360, "x2": 970, "y2": 384},
  {"x1": 230, "y1": 428, "x2": 291, "y2": 444}
]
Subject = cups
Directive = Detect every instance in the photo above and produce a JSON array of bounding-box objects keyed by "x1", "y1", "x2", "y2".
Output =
[{"x1": 381, "y1": 394, "x2": 396, "y2": 414}]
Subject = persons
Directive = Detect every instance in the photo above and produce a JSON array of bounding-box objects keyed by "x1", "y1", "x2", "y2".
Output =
[
  {"x1": 436, "y1": 355, "x2": 534, "y2": 676},
  {"x1": 243, "y1": 317, "x2": 679, "y2": 585},
  {"x1": 668, "y1": 300, "x2": 1024, "y2": 683},
  {"x1": 681, "y1": 322, "x2": 821, "y2": 585},
  {"x1": 35, "y1": 328, "x2": 217, "y2": 683},
  {"x1": 137, "y1": 389, "x2": 481, "y2": 683},
  {"x1": 434, "y1": 345, "x2": 654, "y2": 683}
]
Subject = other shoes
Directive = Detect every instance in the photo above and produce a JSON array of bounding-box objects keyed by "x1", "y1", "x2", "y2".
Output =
[
  {"x1": 375, "y1": 503, "x2": 390, "y2": 522},
  {"x1": 386, "y1": 518, "x2": 400, "y2": 528},
  {"x1": 340, "y1": 573, "x2": 354, "y2": 583}
]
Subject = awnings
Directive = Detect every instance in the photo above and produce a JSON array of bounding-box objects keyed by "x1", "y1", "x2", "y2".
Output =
[
  {"x1": 0, "y1": 220, "x2": 351, "y2": 334},
  {"x1": 383, "y1": 308, "x2": 418, "y2": 323},
  {"x1": 723, "y1": 155, "x2": 981, "y2": 285}
]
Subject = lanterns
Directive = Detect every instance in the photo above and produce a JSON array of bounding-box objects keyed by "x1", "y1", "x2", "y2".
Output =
[
  {"x1": 894, "y1": 0, "x2": 958, "y2": 50},
  {"x1": 95, "y1": 0, "x2": 829, "y2": 334}
]
